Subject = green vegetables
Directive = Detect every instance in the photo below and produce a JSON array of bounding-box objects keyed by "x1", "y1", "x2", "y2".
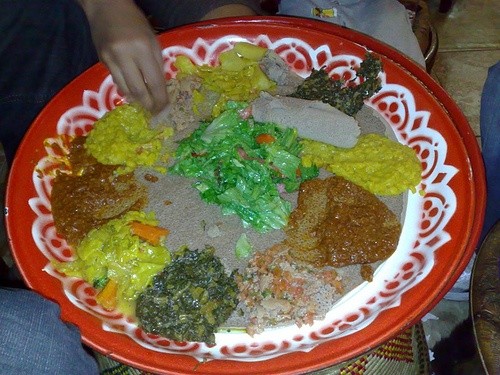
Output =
[{"x1": 166, "y1": 99, "x2": 320, "y2": 257}]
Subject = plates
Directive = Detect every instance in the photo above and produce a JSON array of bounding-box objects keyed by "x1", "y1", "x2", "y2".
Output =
[{"x1": 3, "y1": 15, "x2": 487, "y2": 375}]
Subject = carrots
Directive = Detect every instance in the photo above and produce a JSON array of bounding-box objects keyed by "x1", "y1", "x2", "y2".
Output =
[
  {"x1": 97, "y1": 281, "x2": 117, "y2": 310},
  {"x1": 126, "y1": 221, "x2": 169, "y2": 245}
]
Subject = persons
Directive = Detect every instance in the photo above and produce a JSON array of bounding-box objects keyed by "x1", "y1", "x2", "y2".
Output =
[
  {"x1": 1, "y1": 61, "x2": 500, "y2": 375},
  {"x1": 0, "y1": 0, "x2": 278, "y2": 287}
]
